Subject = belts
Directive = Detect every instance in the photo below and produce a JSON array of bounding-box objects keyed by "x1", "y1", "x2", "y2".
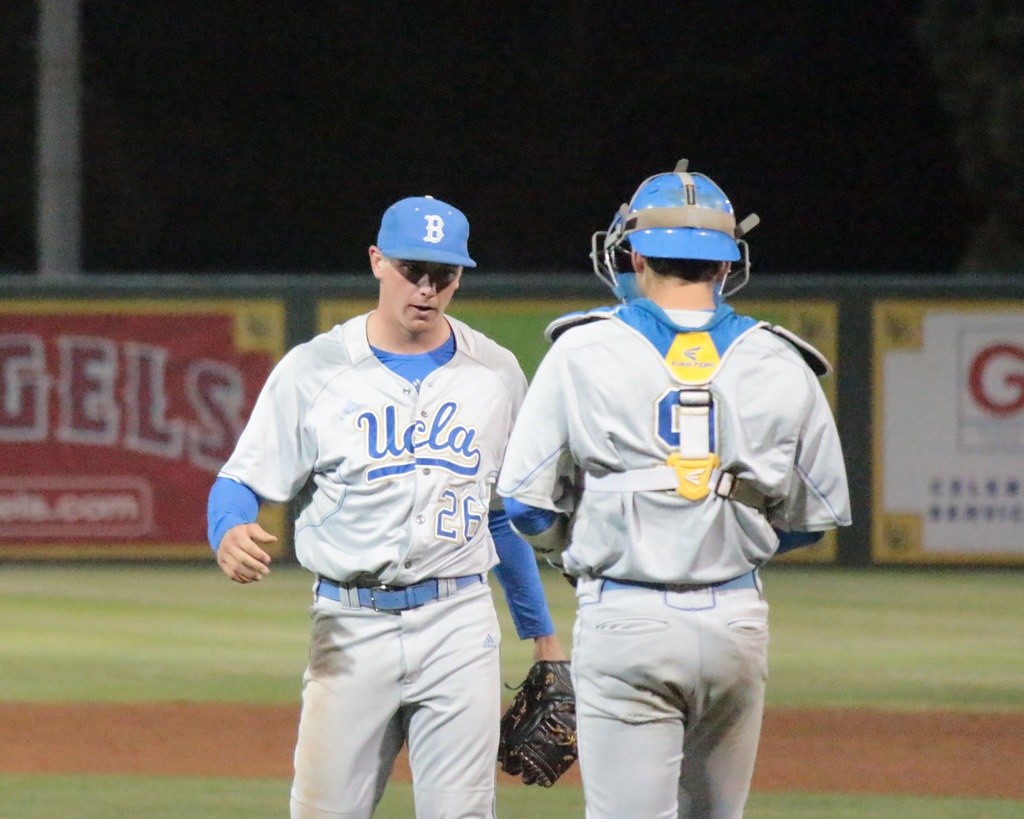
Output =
[
  {"x1": 602, "y1": 569, "x2": 755, "y2": 593},
  {"x1": 317, "y1": 573, "x2": 481, "y2": 611}
]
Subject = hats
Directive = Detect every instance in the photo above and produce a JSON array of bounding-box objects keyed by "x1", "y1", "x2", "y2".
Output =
[{"x1": 377, "y1": 195, "x2": 478, "y2": 267}]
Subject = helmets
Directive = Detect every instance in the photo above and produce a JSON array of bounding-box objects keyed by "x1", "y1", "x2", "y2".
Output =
[{"x1": 626, "y1": 171, "x2": 741, "y2": 260}]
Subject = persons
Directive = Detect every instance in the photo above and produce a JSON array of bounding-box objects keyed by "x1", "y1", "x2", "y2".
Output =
[
  {"x1": 205, "y1": 195, "x2": 565, "y2": 819},
  {"x1": 495, "y1": 158, "x2": 852, "y2": 819}
]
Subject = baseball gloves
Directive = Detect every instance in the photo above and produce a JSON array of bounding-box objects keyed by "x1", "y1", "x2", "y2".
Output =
[{"x1": 495, "y1": 660, "x2": 579, "y2": 789}]
